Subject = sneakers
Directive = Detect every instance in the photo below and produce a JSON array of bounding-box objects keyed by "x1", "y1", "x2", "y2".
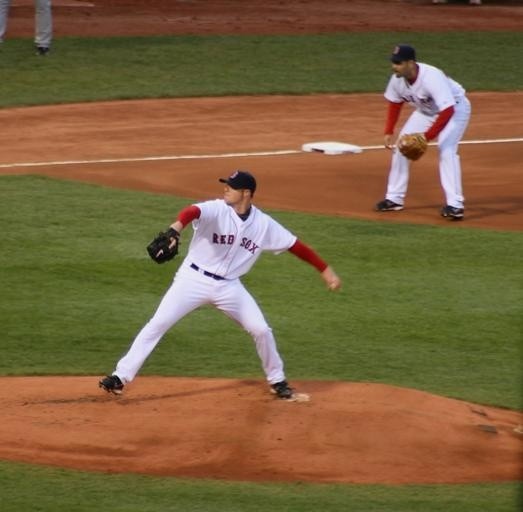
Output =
[
  {"x1": 373, "y1": 199, "x2": 405, "y2": 212},
  {"x1": 440, "y1": 206, "x2": 464, "y2": 219},
  {"x1": 97, "y1": 374, "x2": 124, "y2": 397},
  {"x1": 269, "y1": 379, "x2": 293, "y2": 400}
]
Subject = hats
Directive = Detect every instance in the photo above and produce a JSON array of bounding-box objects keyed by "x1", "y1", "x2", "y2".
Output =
[
  {"x1": 387, "y1": 46, "x2": 416, "y2": 62},
  {"x1": 218, "y1": 170, "x2": 258, "y2": 191}
]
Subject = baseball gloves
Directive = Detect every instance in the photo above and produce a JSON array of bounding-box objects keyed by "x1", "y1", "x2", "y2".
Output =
[
  {"x1": 397, "y1": 133, "x2": 428, "y2": 161},
  {"x1": 147, "y1": 227, "x2": 180, "y2": 264}
]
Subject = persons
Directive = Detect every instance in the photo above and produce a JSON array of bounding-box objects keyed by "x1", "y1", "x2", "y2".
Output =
[
  {"x1": 372, "y1": 44, "x2": 472, "y2": 220},
  {"x1": 0, "y1": 1, "x2": 52, "y2": 56},
  {"x1": 98, "y1": 171, "x2": 341, "y2": 401}
]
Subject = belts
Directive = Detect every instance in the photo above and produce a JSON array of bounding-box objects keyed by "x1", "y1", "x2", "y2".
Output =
[{"x1": 189, "y1": 263, "x2": 231, "y2": 283}]
